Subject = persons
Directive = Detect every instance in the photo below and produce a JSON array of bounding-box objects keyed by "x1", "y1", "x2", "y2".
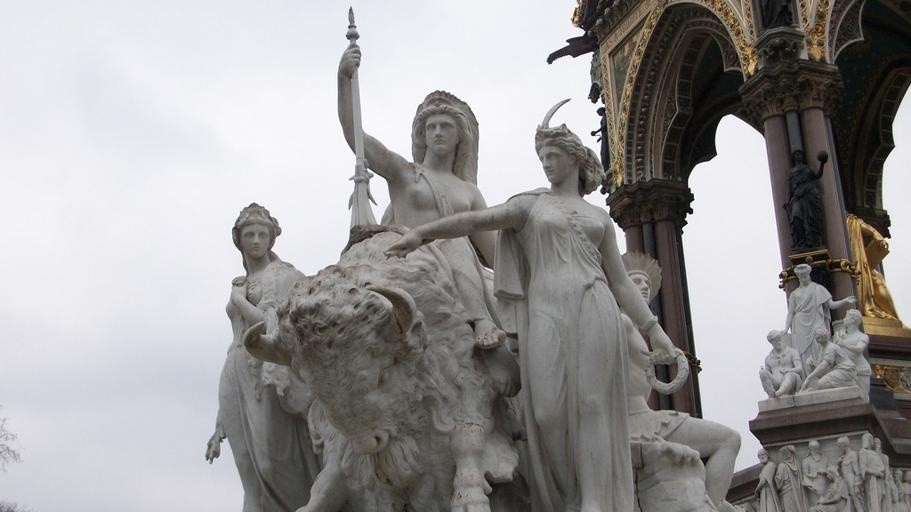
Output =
[
  {"x1": 204, "y1": 203, "x2": 321, "y2": 511},
  {"x1": 381, "y1": 119, "x2": 683, "y2": 512},
  {"x1": 334, "y1": 42, "x2": 507, "y2": 347},
  {"x1": 784, "y1": 146, "x2": 831, "y2": 262},
  {"x1": 617, "y1": 243, "x2": 741, "y2": 512},
  {"x1": 757, "y1": 264, "x2": 903, "y2": 511}
]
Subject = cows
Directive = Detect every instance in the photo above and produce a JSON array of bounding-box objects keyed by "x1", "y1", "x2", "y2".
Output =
[{"x1": 238, "y1": 225, "x2": 524, "y2": 512}]
